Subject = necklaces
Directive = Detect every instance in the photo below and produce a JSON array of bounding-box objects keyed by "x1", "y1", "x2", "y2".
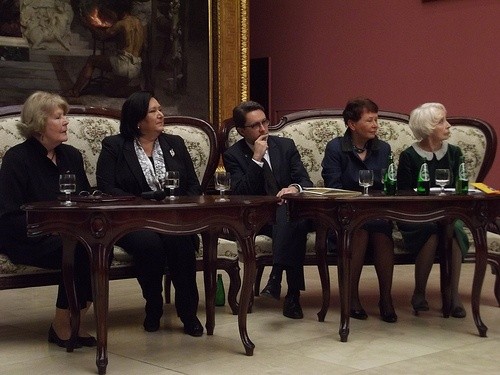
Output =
[
  {"x1": 353, "y1": 143, "x2": 367, "y2": 153},
  {"x1": 51, "y1": 154, "x2": 54, "y2": 160}
]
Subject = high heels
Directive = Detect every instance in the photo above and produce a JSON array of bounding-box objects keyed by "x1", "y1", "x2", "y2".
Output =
[
  {"x1": 350, "y1": 306, "x2": 368, "y2": 320},
  {"x1": 378, "y1": 300, "x2": 397, "y2": 323},
  {"x1": 443, "y1": 299, "x2": 466, "y2": 318},
  {"x1": 411, "y1": 297, "x2": 429, "y2": 316}
]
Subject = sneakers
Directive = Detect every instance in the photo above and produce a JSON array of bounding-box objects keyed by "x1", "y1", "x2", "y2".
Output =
[
  {"x1": 261, "y1": 277, "x2": 281, "y2": 300},
  {"x1": 283, "y1": 294, "x2": 304, "y2": 319}
]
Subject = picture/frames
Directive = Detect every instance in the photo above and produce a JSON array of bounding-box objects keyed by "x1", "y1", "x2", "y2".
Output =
[{"x1": 250, "y1": 56, "x2": 271, "y2": 124}]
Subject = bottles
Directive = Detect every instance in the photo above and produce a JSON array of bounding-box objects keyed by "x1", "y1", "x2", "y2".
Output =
[
  {"x1": 455, "y1": 156, "x2": 469, "y2": 195},
  {"x1": 417, "y1": 157, "x2": 431, "y2": 195},
  {"x1": 216, "y1": 274, "x2": 225, "y2": 306},
  {"x1": 383, "y1": 155, "x2": 398, "y2": 196}
]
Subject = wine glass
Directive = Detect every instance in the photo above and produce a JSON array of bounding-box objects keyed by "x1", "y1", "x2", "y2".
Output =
[
  {"x1": 165, "y1": 171, "x2": 180, "y2": 199},
  {"x1": 58, "y1": 174, "x2": 78, "y2": 205},
  {"x1": 358, "y1": 170, "x2": 375, "y2": 198},
  {"x1": 434, "y1": 169, "x2": 451, "y2": 196},
  {"x1": 214, "y1": 172, "x2": 231, "y2": 202}
]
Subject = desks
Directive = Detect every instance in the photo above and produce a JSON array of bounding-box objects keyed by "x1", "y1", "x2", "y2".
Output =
[
  {"x1": 21, "y1": 195, "x2": 280, "y2": 375},
  {"x1": 281, "y1": 190, "x2": 500, "y2": 343}
]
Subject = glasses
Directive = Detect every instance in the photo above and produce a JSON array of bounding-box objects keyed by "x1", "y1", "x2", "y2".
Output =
[{"x1": 242, "y1": 120, "x2": 269, "y2": 130}]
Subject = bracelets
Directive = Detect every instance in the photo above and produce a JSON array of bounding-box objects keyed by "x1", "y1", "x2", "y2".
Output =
[{"x1": 294, "y1": 185, "x2": 299, "y2": 190}]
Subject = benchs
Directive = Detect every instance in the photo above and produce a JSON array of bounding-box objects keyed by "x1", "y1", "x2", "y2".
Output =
[
  {"x1": 227, "y1": 109, "x2": 500, "y2": 296},
  {"x1": 1, "y1": 105, "x2": 219, "y2": 305}
]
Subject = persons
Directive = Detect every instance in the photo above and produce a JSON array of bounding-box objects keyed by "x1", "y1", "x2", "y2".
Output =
[
  {"x1": 222, "y1": 101, "x2": 313, "y2": 319},
  {"x1": 320, "y1": 97, "x2": 397, "y2": 323},
  {"x1": 96, "y1": 92, "x2": 204, "y2": 337},
  {"x1": 0, "y1": 90, "x2": 98, "y2": 348},
  {"x1": 395, "y1": 103, "x2": 466, "y2": 318}
]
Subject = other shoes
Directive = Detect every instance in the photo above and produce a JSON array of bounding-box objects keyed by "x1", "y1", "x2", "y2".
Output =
[
  {"x1": 47, "y1": 324, "x2": 82, "y2": 348},
  {"x1": 144, "y1": 308, "x2": 163, "y2": 332},
  {"x1": 78, "y1": 335, "x2": 96, "y2": 346},
  {"x1": 180, "y1": 315, "x2": 203, "y2": 336}
]
